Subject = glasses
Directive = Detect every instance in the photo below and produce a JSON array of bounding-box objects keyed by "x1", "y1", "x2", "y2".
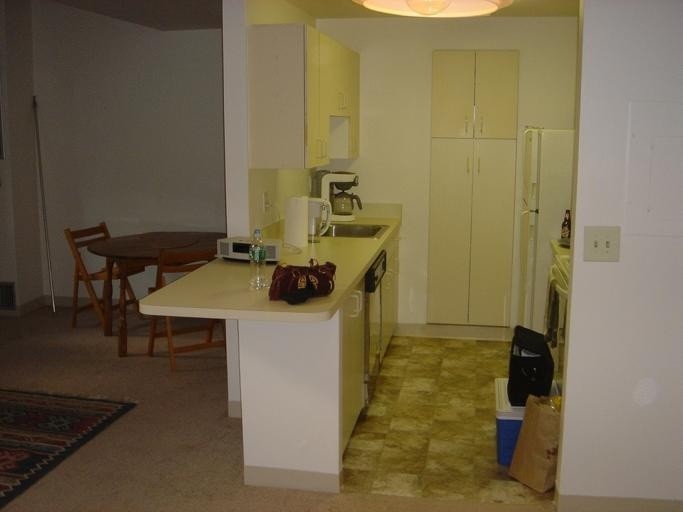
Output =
[{"x1": 353, "y1": 0, "x2": 516, "y2": 20}]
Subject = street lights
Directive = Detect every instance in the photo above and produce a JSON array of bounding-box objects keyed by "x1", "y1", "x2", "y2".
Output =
[
  {"x1": 508, "y1": 325, "x2": 553, "y2": 406},
  {"x1": 508, "y1": 395, "x2": 560, "y2": 494},
  {"x1": 269, "y1": 261, "x2": 336, "y2": 304}
]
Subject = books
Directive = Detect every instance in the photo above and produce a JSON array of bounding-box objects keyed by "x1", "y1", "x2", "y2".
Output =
[{"x1": 493, "y1": 375, "x2": 560, "y2": 472}]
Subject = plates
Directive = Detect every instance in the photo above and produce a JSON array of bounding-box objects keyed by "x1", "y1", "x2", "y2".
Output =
[
  {"x1": 429, "y1": 141, "x2": 512, "y2": 333},
  {"x1": 331, "y1": 51, "x2": 361, "y2": 160},
  {"x1": 303, "y1": 23, "x2": 332, "y2": 168},
  {"x1": 237, "y1": 271, "x2": 364, "y2": 493},
  {"x1": 430, "y1": 51, "x2": 520, "y2": 141},
  {"x1": 379, "y1": 220, "x2": 401, "y2": 378},
  {"x1": 331, "y1": 38, "x2": 352, "y2": 117}
]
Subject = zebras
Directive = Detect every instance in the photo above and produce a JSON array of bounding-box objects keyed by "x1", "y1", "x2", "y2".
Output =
[{"x1": 543, "y1": 265, "x2": 570, "y2": 397}]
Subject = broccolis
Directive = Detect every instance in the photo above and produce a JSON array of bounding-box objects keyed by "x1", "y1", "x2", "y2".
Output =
[{"x1": 516, "y1": 128, "x2": 580, "y2": 335}]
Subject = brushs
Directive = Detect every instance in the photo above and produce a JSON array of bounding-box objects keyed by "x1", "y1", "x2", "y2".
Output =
[{"x1": 310, "y1": 170, "x2": 362, "y2": 222}]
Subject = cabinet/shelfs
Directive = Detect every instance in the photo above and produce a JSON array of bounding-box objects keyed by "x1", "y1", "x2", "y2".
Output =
[
  {"x1": 249, "y1": 229, "x2": 267, "y2": 289},
  {"x1": 561, "y1": 209, "x2": 570, "y2": 238}
]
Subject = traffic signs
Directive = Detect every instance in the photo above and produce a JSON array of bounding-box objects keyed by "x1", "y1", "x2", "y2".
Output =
[{"x1": 262, "y1": 190, "x2": 271, "y2": 216}]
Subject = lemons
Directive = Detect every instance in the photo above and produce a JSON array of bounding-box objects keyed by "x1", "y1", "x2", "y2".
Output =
[{"x1": 308, "y1": 198, "x2": 331, "y2": 243}]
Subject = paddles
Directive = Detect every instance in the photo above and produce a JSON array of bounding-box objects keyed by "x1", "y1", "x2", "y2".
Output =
[{"x1": 1, "y1": 385, "x2": 141, "y2": 508}]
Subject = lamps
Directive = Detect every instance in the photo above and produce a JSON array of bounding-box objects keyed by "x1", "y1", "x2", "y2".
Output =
[{"x1": 63, "y1": 221, "x2": 226, "y2": 368}]
